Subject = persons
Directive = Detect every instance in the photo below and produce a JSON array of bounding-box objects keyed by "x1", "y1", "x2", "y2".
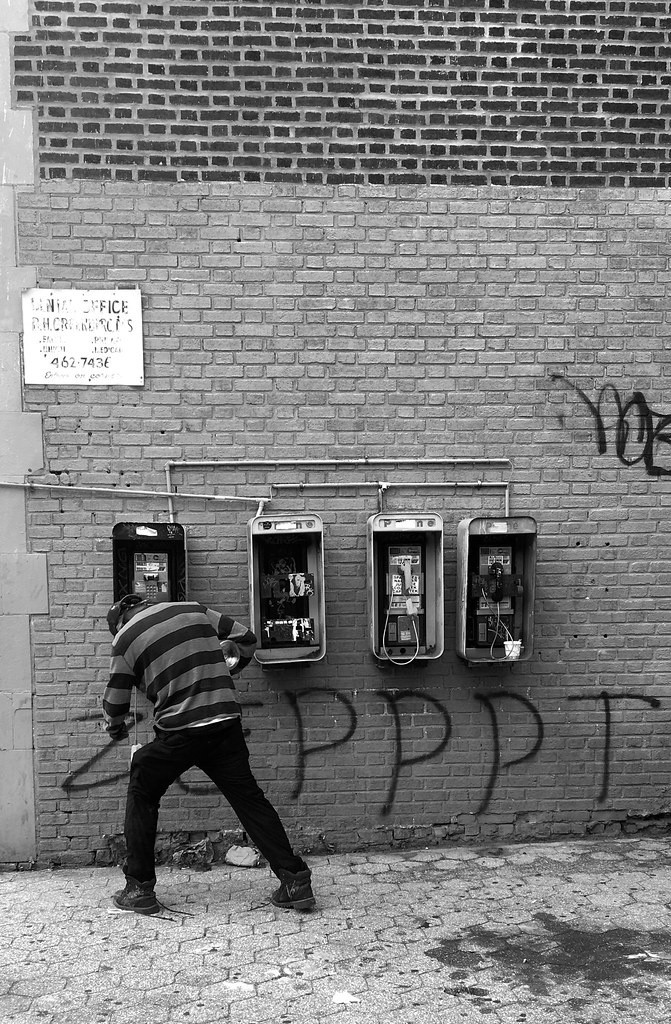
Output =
[{"x1": 102, "y1": 595, "x2": 317, "y2": 914}]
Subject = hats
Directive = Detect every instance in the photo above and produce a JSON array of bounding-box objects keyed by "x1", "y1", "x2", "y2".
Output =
[{"x1": 106, "y1": 594, "x2": 145, "y2": 639}]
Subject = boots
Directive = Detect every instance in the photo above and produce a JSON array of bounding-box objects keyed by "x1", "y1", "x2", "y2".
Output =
[
  {"x1": 112, "y1": 863, "x2": 160, "y2": 914},
  {"x1": 270, "y1": 867, "x2": 316, "y2": 910}
]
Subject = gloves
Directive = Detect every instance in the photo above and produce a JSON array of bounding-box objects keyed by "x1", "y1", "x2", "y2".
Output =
[{"x1": 106, "y1": 721, "x2": 130, "y2": 742}]
